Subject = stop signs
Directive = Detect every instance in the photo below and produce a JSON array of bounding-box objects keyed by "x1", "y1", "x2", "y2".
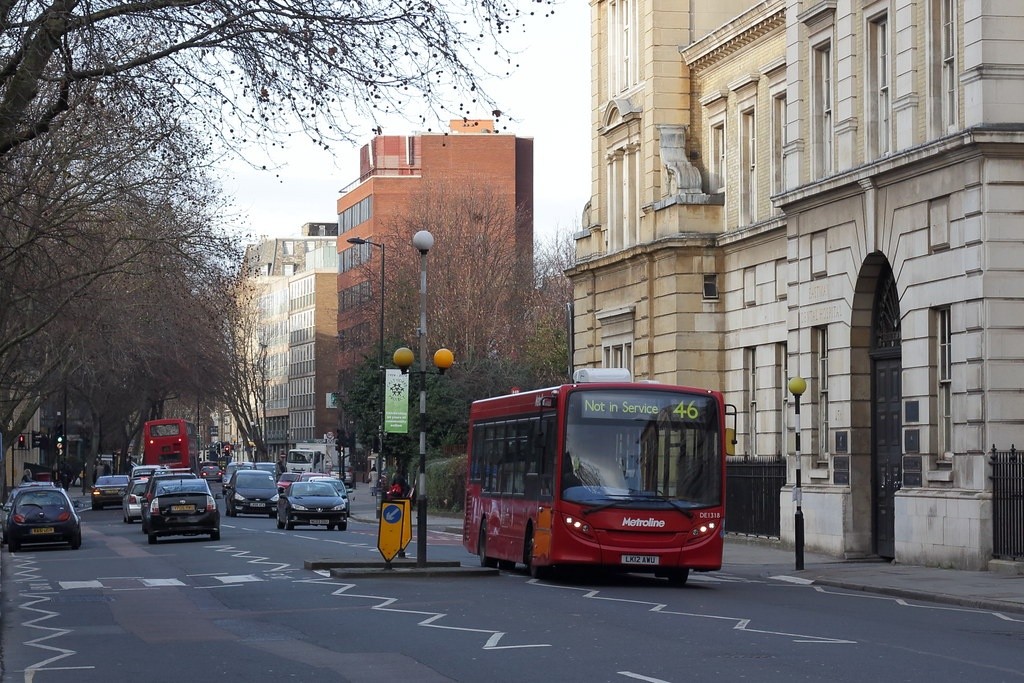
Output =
[{"x1": 281, "y1": 453, "x2": 286, "y2": 459}]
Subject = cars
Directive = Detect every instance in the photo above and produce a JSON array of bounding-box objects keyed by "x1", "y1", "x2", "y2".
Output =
[
  {"x1": 277, "y1": 473, "x2": 353, "y2": 517},
  {"x1": 117, "y1": 467, "x2": 198, "y2": 523},
  {"x1": 201, "y1": 466, "x2": 223, "y2": 482},
  {"x1": 0, "y1": 482, "x2": 81, "y2": 551},
  {"x1": 140, "y1": 478, "x2": 223, "y2": 544},
  {"x1": 276, "y1": 481, "x2": 347, "y2": 532},
  {"x1": 91, "y1": 475, "x2": 130, "y2": 511},
  {"x1": 224, "y1": 470, "x2": 280, "y2": 519},
  {"x1": 221, "y1": 462, "x2": 280, "y2": 493}
]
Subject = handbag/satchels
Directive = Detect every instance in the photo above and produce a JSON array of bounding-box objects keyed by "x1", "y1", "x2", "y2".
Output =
[{"x1": 368, "y1": 472, "x2": 371, "y2": 479}]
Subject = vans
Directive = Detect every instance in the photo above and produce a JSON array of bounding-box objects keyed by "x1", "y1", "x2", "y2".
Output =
[{"x1": 85, "y1": 456, "x2": 140, "y2": 474}]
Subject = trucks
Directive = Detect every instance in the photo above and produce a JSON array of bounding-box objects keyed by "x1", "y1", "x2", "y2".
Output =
[{"x1": 285, "y1": 441, "x2": 337, "y2": 472}]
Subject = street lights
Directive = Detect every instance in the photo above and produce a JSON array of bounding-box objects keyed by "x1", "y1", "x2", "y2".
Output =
[
  {"x1": 347, "y1": 238, "x2": 386, "y2": 521},
  {"x1": 788, "y1": 377, "x2": 807, "y2": 571},
  {"x1": 392, "y1": 230, "x2": 454, "y2": 564}
]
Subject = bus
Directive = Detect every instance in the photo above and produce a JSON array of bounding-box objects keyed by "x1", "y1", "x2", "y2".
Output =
[
  {"x1": 144, "y1": 419, "x2": 198, "y2": 469},
  {"x1": 462, "y1": 366, "x2": 737, "y2": 580}
]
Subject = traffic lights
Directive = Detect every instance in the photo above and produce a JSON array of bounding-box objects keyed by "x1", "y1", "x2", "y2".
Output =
[
  {"x1": 224, "y1": 445, "x2": 230, "y2": 457},
  {"x1": 18, "y1": 436, "x2": 25, "y2": 447},
  {"x1": 34, "y1": 435, "x2": 42, "y2": 448},
  {"x1": 216, "y1": 443, "x2": 221, "y2": 455}
]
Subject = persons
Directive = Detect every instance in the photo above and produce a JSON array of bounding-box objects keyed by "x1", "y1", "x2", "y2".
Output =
[
  {"x1": 92, "y1": 459, "x2": 111, "y2": 485},
  {"x1": 19, "y1": 469, "x2": 37, "y2": 483},
  {"x1": 368, "y1": 467, "x2": 408, "y2": 498}
]
